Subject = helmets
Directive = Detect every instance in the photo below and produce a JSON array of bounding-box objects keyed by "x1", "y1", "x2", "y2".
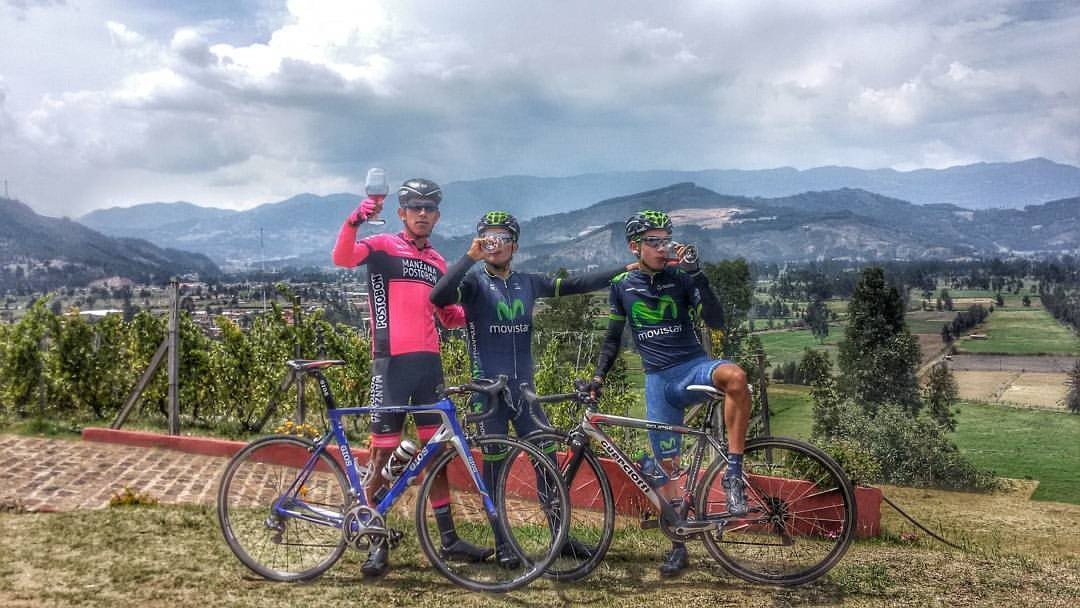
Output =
[
  {"x1": 624, "y1": 209, "x2": 673, "y2": 241},
  {"x1": 477, "y1": 210, "x2": 520, "y2": 243},
  {"x1": 397, "y1": 179, "x2": 442, "y2": 209}
]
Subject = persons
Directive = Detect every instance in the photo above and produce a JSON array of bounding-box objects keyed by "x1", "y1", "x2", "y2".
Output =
[
  {"x1": 428, "y1": 212, "x2": 641, "y2": 570},
  {"x1": 576, "y1": 211, "x2": 750, "y2": 578},
  {"x1": 331, "y1": 179, "x2": 497, "y2": 576}
]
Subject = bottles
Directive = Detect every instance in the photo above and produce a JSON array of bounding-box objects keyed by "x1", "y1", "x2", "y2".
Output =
[
  {"x1": 381, "y1": 439, "x2": 418, "y2": 482},
  {"x1": 636, "y1": 454, "x2": 679, "y2": 505}
]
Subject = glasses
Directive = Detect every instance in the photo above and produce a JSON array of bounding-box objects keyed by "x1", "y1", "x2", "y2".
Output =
[
  {"x1": 479, "y1": 231, "x2": 513, "y2": 241},
  {"x1": 641, "y1": 234, "x2": 672, "y2": 248},
  {"x1": 403, "y1": 201, "x2": 438, "y2": 212}
]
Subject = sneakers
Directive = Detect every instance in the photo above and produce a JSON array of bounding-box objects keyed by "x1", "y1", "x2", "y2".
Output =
[
  {"x1": 659, "y1": 545, "x2": 690, "y2": 576},
  {"x1": 721, "y1": 472, "x2": 749, "y2": 518},
  {"x1": 438, "y1": 539, "x2": 495, "y2": 562},
  {"x1": 551, "y1": 535, "x2": 595, "y2": 558},
  {"x1": 360, "y1": 540, "x2": 389, "y2": 576},
  {"x1": 496, "y1": 538, "x2": 520, "y2": 569}
]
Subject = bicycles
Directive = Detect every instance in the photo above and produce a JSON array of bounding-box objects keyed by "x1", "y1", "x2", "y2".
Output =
[
  {"x1": 215, "y1": 359, "x2": 573, "y2": 597},
  {"x1": 495, "y1": 376, "x2": 858, "y2": 587}
]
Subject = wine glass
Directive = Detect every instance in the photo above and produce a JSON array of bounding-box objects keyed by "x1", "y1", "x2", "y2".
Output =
[
  {"x1": 481, "y1": 232, "x2": 504, "y2": 253},
  {"x1": 663, "y1": 240, "x2": 698, "y2": 264},
  {"x1": 364, "y1": 167, "x2": 390, "y2": 225}
]
